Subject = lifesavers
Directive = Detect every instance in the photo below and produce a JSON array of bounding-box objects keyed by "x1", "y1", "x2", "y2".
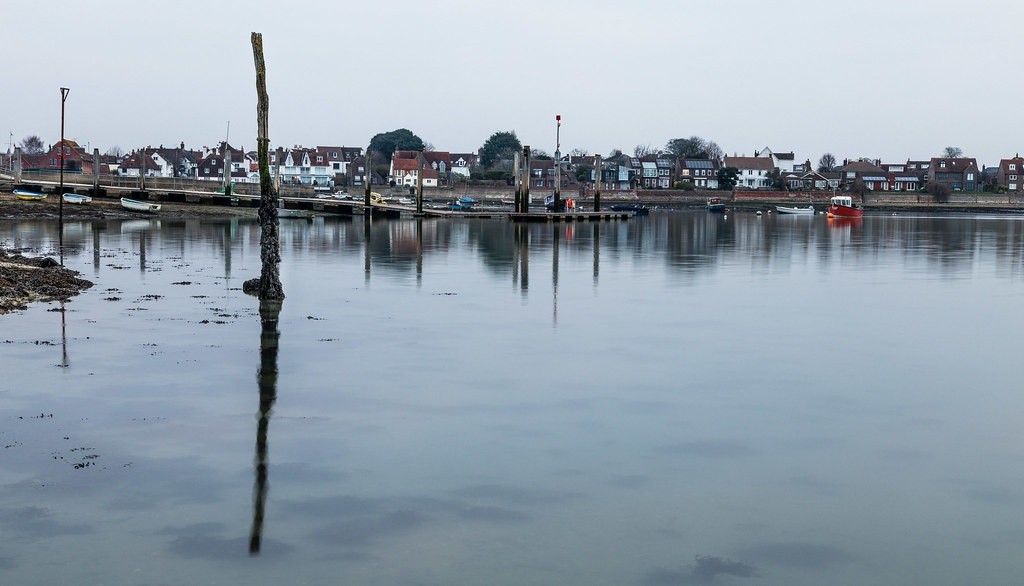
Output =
[{"x1": 568, "y1": 199, "x2": 573, "y2": 208}]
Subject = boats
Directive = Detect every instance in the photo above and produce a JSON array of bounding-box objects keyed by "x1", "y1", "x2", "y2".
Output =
[
  {"x1": 364, "y1": 192, "x2": 388, "y2": 206},
  {"x1": 332, "y1": 191, "x2": 353, "y2": 201},
  {"x1": 63, "y1": 192, "x2": 92, "y2": 205},
  {"x1": 500, "y1": 194, "x2": 515, "y2": 203},
  {"x1": 776, "y1": 206, "x2": 815, "y2": 214},
  {"x1": 396, "y1": 198, "x2": 416, "y2": 205},
  {"x1": 828, "y1": 196, "x2": 863, "y2": 218},
  {"x1": 121, "y1": 197, "x2": 161, "y2": 213},
  {"x1": 705, "y1": 197, "x2": 725, "y2": 210},
  {"x1": 448, "y1": 197, "x2": 479, "y2": 207},
  {"x1": 543, "y1": 195, "x2": 565, "y2": 210},
  {"x1": 13, "y1": 189, "x2": 47, "y2": 200},
  {"x1": 610, "y1": 204, "x2": 650, "y2": 214}
]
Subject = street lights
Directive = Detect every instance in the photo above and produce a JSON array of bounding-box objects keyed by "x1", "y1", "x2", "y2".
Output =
[
  {"x1": 10, "y1": 130, "x2": 14, "y2": 172},
  {"x1": 556, "y1": 114, "x2": 561, "y2": 205}
]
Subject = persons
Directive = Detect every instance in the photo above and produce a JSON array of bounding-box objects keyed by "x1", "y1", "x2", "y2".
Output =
[
  {"x1": 312, "y1": 180, "x2": 318, "y2": 192},
  {"x1": 390, "y1": 178, "x2": 396, "y2": 187},
  {"x1": 567, "y1": 197, "x2": 572, "y2": 212}
]
